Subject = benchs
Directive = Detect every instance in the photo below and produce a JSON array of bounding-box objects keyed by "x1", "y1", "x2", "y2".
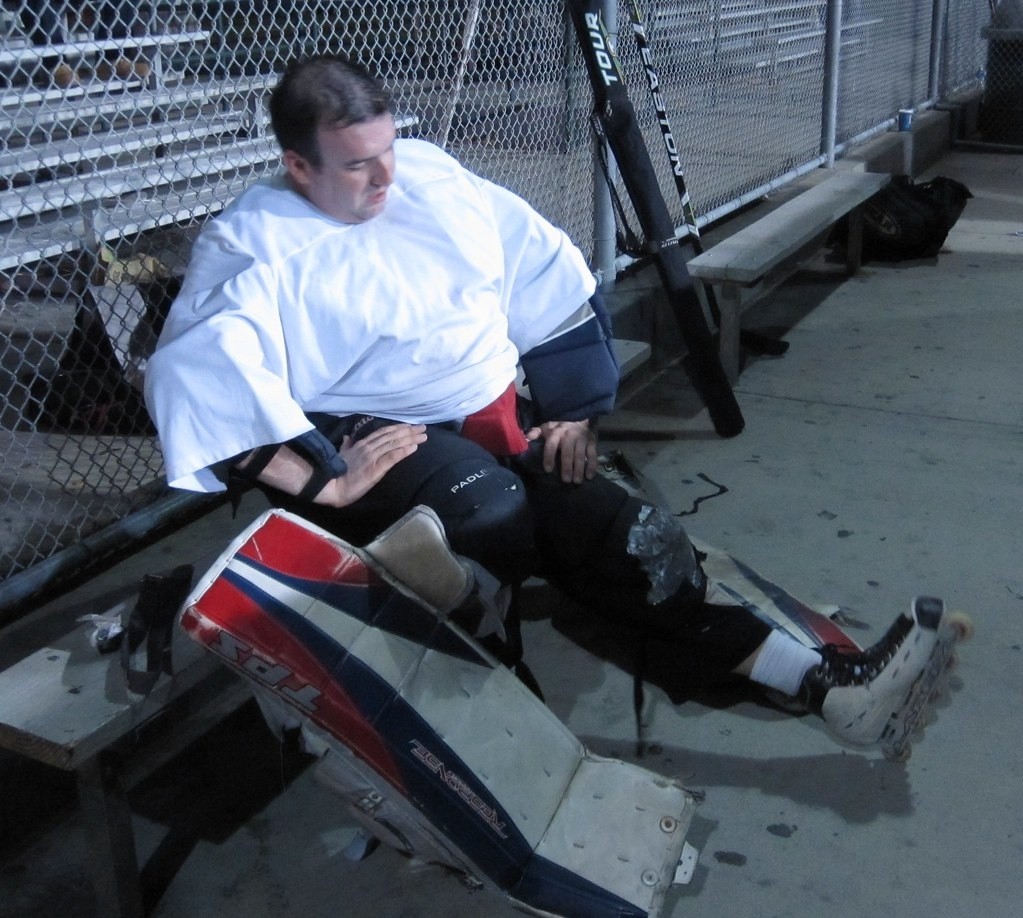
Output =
[
  {"x1": 688, "y1": 167, "x2": 893, "y2": 382},
  {"x1": 0, "y1": 10, "x2": 418, "y2": 276},
  {"x1": 0, "y1": 337, "x2": 653, "y2": 918},
  {"x1": 647, "y1": 0, "x2": 886, "y2": 84}
]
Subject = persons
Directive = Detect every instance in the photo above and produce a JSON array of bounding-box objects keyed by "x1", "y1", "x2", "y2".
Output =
[{"x1": 143, "y1": 52, "x2": 976, "y2": 918}]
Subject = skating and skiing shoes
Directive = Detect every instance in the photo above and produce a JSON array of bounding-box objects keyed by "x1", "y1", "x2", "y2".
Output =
[{"x1": 802, "y1": 597, "x2": 975, "y2": 765}]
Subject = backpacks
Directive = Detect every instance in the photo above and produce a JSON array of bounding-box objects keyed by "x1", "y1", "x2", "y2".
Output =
[{"x1": 828, "y1": 171, "x2": 973, "y2": 261}]
